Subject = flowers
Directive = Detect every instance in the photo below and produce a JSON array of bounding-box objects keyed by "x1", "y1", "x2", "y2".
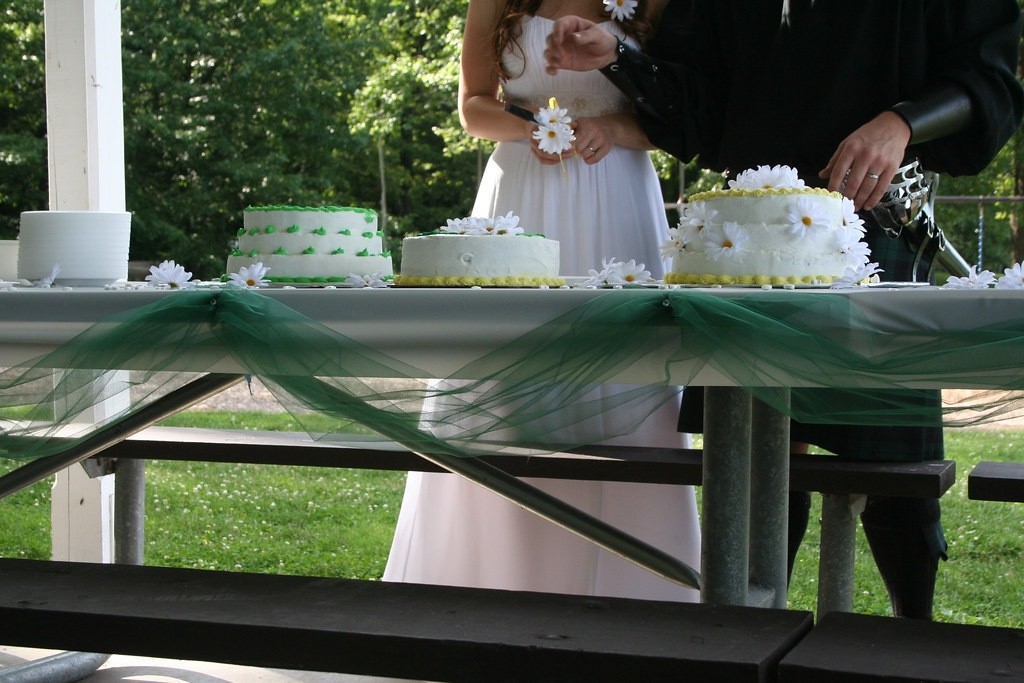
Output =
[
  {"x1": 144, "y1": 259, "x2": 194, "y2": 288},
  {"x1": 226, "y1": 262, "x2": 274, "y2": 289},
  {"x1": 346, "y1": 271, "x2": 387, "y2": 289},
  {"x1": 438, "y1": 210, "x2": 526, "y2": 237},
  {"x1": 726, "y1": 163, "x2": 812, "y2": 193},
  {"x1": 995, "y1": 259, "x2": 1024, "y2": 290},
  {"x1": 785, "y1": 197, "x2": 828, "y2": 239},
  {"x1": 34, "y1": 263, "x2": 62, "y2": 288},
  {"x1": 532, "y1": 103, "x2": 580, "y2": 184},
  {"x1": 658, "y1": 201, "x2": 750, "y2": 261},
  {"x1": 581, "y1": 259, "x2": 653, "y2": 288},
  {"x1": 602, "y1": 0, "x2": 639, "y2": 22},
  {"x1": 940, "y1": 264, "x2": 998, "y2": 289},
  {"x1": 831, "y1": 196, "x2": 887, "y2": 290}
]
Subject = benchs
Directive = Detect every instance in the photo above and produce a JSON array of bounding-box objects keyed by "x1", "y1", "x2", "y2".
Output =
[{"x1": 1, "y1": 417, "x2": 1024, "y2": 683}]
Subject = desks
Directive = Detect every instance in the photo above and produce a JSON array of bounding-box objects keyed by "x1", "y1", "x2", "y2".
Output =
[{"x1": 0, "y1": 280, "x2": 1024, "y2": 615}]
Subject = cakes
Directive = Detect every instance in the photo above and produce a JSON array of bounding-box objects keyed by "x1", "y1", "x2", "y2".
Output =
[
  {"x1": 391, "y1": 210, "x2": 567, "y2": 286},
  {"x1": 220, "y1": 204, "x2": 396, "y2": 284},
  {"x1": 661, "y1": 164, "x2": 884, "y2": 286}
]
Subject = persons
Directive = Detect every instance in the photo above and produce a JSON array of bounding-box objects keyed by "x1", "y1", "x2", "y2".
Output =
[
  {"x1": 544, "y1": 0, "x2": 1024, "y2": 622},
  {"x1": 380, "y1": 0, "x2": 701, "y2": 604}
]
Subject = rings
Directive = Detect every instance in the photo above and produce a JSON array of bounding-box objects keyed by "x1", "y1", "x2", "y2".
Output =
[
  {"x1": 587, "y1": 146, "x2": 596, "y2": 152},
  {"x1": 867, "y1": 173, "x2": 879, "y2": 179}
]
